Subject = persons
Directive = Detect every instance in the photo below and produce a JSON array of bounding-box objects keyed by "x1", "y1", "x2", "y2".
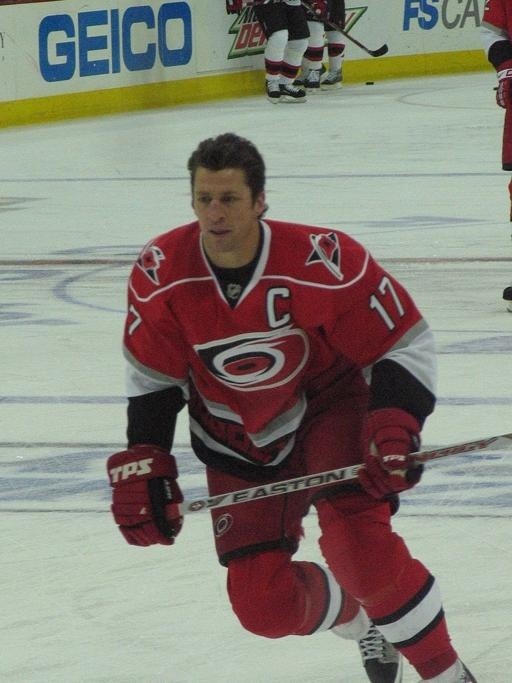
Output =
[
  {"x1": 482, "y1": 0, "x2": 512, "y2": 301},
  {"x1": 296, "y1": 0, "x2": 345, "y2": 88},
  {"x1": 108, "y1": 132, "x2": 478, "y2": 682},
  {"x1": 225, "y1": 0, "x2": 329, "y2": 99}
]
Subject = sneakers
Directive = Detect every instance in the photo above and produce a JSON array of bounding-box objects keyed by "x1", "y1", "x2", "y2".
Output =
[
  {"x1": 305, "y1": 70, "x2": 327, "y2": 88},
  {"x1": 355, "y1": 618, "x2": 402, "y2": 683},
  {"x1": 321, "y1": 68, "x2": 343, "y2": 84},
  {"x1": 279, "y1": 83, "x2": 305, "y2": 98},
  {"x1": 265, "y1": 80, "x2": 282, "y2": 98}
]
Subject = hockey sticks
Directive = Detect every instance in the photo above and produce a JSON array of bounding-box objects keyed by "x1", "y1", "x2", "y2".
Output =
[{"x1": 301, "y1": 2, "x2": 388, "y2": 56}]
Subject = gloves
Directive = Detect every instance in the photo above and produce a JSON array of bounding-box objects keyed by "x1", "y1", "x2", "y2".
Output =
[
  {"x1": 493, "y1": 60, "x2": 512, "y2": 111},
  {"x1": 353, "y1": 407, "x2": 423, "y2": 498},
  {"x1": 105, "y1": 447, "x2": 183, "y2": 546},
  {"x1": 312, "y1": 1, "x2": 328, "y2": 18}
]
